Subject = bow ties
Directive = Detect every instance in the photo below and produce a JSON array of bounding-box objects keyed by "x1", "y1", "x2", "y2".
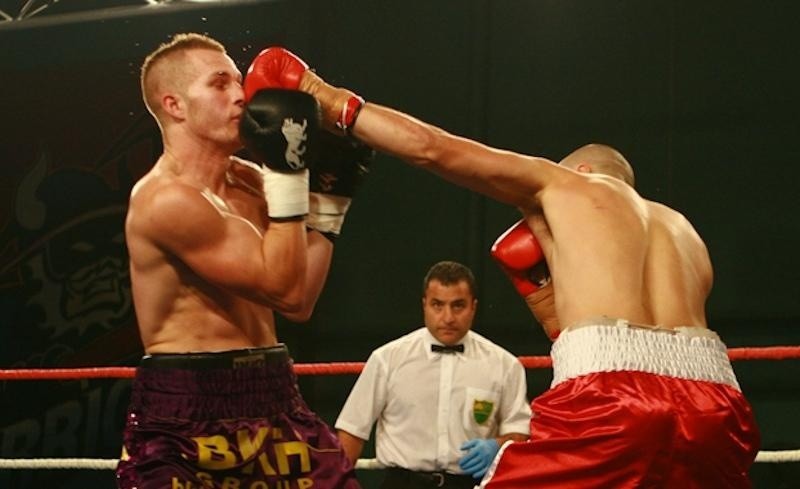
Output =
[{"x1": 431, "y1": 345, "x2": 464, "y2": 352}]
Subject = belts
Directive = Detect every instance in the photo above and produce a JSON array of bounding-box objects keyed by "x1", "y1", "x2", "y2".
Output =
[{"x1": 399, "y1": 471, "x2": 472, "y2": 488}]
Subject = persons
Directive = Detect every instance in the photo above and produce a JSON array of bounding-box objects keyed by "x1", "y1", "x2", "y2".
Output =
[
  {"x1": 243, "y1": 45, "x2": 759, "y2": 489},
  {"x1": 112, "y1": 32, "x2": 376, "y2": 489},
  {"x1": 333, "y1": 261, "x2": 532, "y2": 489}
]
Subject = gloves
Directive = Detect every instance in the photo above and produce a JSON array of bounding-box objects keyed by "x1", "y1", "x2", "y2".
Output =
[
  {"x1": 491, "y1": 216, "x2": 561, "y2": 341},
  {"x1": 458, "y1": 440, "x2": 500, "y2": 478},
  {"x1": 307, "y1": 131, "x2": 375, "y2": 242},
  {"x1": 239, "y1": 88, "x2": 322, "y2": 222},
  {"x1": 244, "y1": 48, "x2": 365, "y2": 136}
]
